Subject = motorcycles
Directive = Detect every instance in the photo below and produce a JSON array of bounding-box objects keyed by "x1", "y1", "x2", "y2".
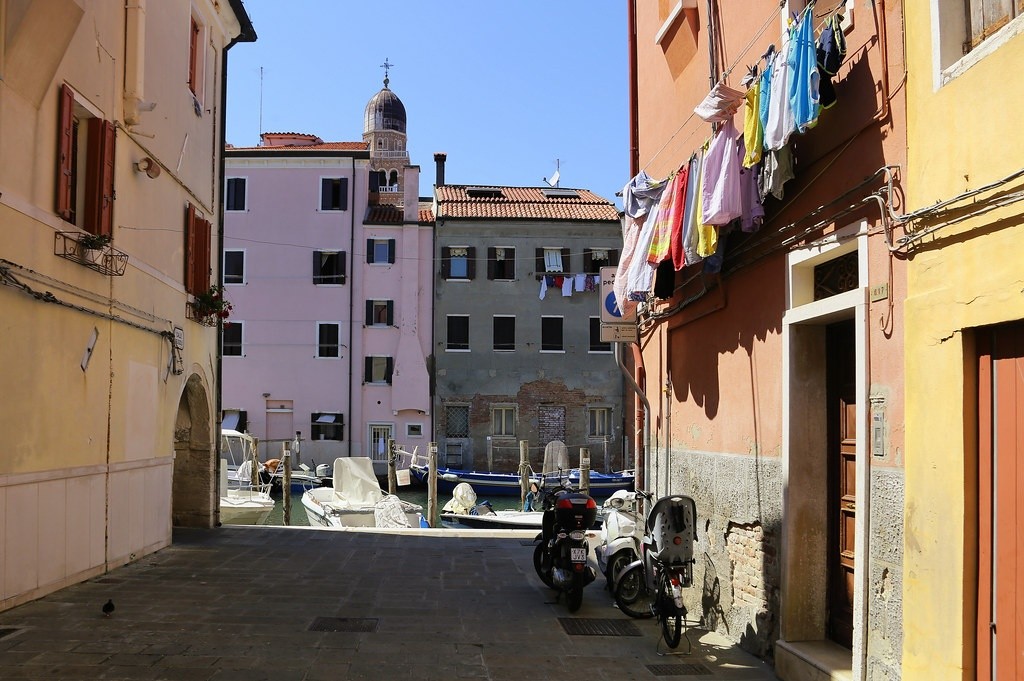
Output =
[
  {"x1": 531, "y1": 464, "x2": 597, "y2": 612},
  {"x1": 592, "y1": 488, "x2": 655, "y2": 605}
]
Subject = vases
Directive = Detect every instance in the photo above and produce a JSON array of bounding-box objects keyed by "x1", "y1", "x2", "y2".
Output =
[
  {"x1": 193, "y1": 309, "x2": 205, "y2": 322},
  {"x1": 84, "y1": 249, "x2": 104, "y2": 265}
]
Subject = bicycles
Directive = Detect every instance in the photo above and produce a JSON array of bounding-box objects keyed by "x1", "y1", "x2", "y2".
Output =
[{"x1": 613, "y1": 486, "x2": 698, "y2": 657}]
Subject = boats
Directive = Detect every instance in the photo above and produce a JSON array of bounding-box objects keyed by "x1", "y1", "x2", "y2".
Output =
[
  {"x1": 300, "y1": 455, "x2": 430, "y2": 528},
  {"x1": 408, "y1": 458, "x2": 635, "y2": 499},
  {"x1": 220, "y1": 427, "x2": 276, "y2": 526},
  {"x1": 258, "y1": 458, "x2": 333, "y2": 492},
  {"x1": 438, "y1": 489, "x2": 636, "y2": 529}
]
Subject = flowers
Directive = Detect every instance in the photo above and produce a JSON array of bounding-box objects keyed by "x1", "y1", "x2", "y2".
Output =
[
  {"x1": 77, "y1": 233, "x2": 115, "y2": 250},
  {"x1": 192, "y1": 284, "x2": 236, "y2": 329}
]
